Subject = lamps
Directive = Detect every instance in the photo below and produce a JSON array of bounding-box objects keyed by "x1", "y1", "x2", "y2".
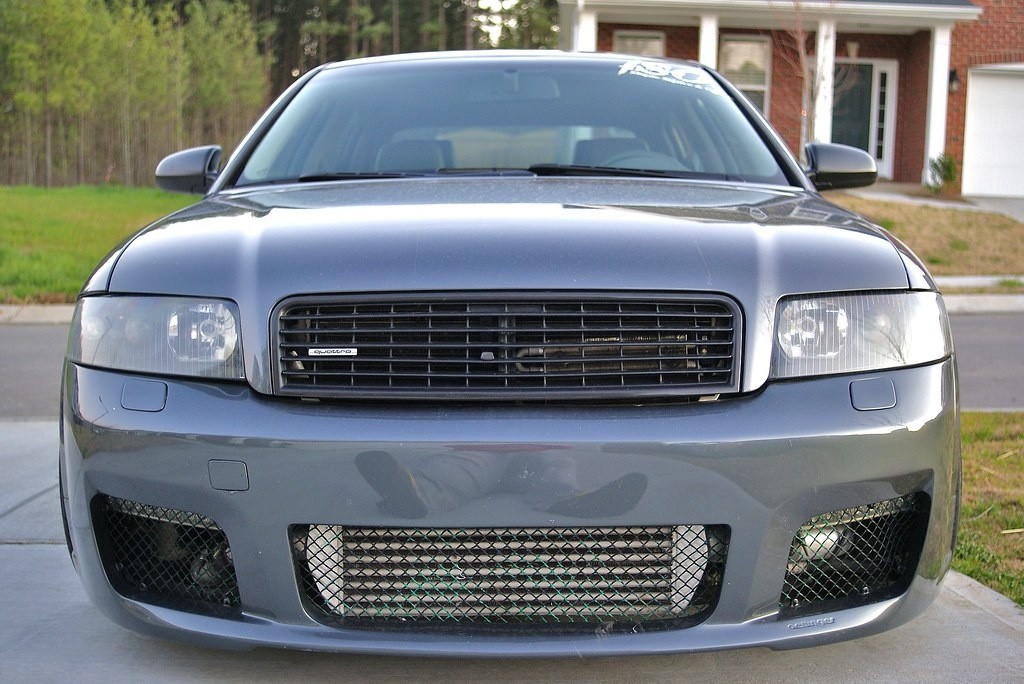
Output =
[{"x1": 949, "y1": 68, "x2": 962, "y2": 94}]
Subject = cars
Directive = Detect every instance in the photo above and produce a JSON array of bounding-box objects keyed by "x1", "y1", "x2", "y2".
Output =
[{"x1": 58, "y1": 49, "x2": 962, "y2": 661}]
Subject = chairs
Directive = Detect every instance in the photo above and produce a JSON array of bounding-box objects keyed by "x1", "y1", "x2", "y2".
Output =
[
  {"x1": 572, "y1": 137, "x2": 649, "y2": 166},
  {"x1": 373, "y1": 142, "x2": 444, "y2": 172}
]
